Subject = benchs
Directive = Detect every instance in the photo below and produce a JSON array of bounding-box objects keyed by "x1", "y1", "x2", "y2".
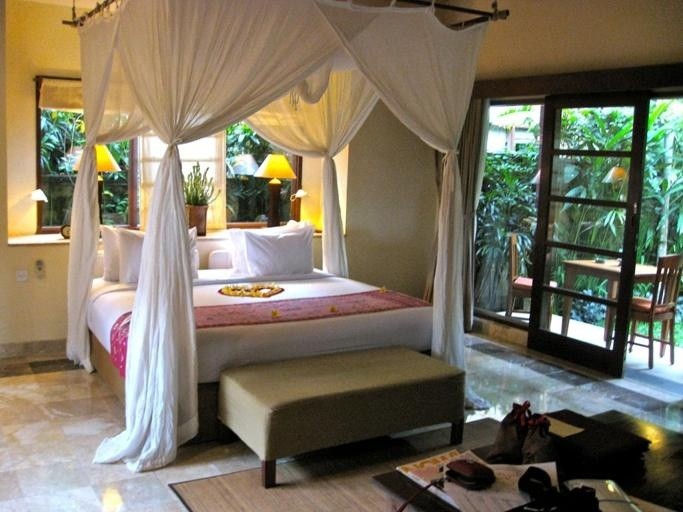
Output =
[{"x1": 219, "y1": 347, "x2": 466, "y2": 490}]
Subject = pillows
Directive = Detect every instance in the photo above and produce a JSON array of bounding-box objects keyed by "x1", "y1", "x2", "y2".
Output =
[
  {"x1": 100, "y1": 226, "x2": 199, "y2": 286},
  {"x1": 226, "y1": 222, "x2": 314, "y2": 277}
]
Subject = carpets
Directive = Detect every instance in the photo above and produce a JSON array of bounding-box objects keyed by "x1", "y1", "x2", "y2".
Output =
[{"x1": 168, "y1": 417, "x2": 503, "y2": 512}]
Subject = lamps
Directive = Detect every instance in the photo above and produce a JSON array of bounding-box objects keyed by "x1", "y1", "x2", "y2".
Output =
[
  {"x1": 253, "y1": 154, "x2": 297, "y2": 227},
  {"x1": 228, "y1": 155, "x2": 259, "y2": 222},
  {"x1": 604, "y1": 138, "x2": 630, "y2": 195},
  {"x1": 72, "y1": 144, "x2": 121, "y2": 237}
]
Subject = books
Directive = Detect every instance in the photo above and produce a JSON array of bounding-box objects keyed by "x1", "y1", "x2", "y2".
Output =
[
  {"x1": 563, "y1": 478, "x2": 639, "y2": 512},
  {"x1": 444, "y1": 461, "x2": 560, "y2": 511},
  {"x1": 395, "y1": 449, "x2": 457, "y2": 508},
  {"x1": 411, "y1": 451, "x2": 489, "y2": 509}
]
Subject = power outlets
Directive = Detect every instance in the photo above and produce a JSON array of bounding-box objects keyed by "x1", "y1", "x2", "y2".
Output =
[{"x1": 17, "y1": 270, "x2": 28, "y2": 281}]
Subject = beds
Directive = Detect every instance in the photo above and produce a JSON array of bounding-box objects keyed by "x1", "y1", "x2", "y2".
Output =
[{"x1": 87, "y1": 237, "x2": 433, "y2": 450}]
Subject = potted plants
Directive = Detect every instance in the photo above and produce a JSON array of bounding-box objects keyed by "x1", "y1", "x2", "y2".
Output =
[{"x1": 183, "y1": 162, "x2": 220, "y2": 236}]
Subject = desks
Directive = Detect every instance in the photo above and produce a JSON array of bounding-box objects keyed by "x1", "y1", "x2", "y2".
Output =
[{"x1": 562, "y1": 260, "x2": 678, "y2": 349}]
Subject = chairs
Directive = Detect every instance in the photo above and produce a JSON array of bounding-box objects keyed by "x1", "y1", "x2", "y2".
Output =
[
  {"x1": 505, "y1": 235, "x2": 558, "y2": 330},
  {"x1": 606, "y1": 255, "x2": 682, "y2": 370}
]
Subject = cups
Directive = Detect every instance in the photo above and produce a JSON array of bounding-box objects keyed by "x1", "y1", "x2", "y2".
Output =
[{"x1": 594, "y1": 247, "x2": 604, "y2": 263}]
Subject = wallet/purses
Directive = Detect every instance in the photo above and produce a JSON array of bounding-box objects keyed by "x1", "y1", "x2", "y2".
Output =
[{"x1": 446, "y1": 458, "x2": 494, "y2": 491}]
